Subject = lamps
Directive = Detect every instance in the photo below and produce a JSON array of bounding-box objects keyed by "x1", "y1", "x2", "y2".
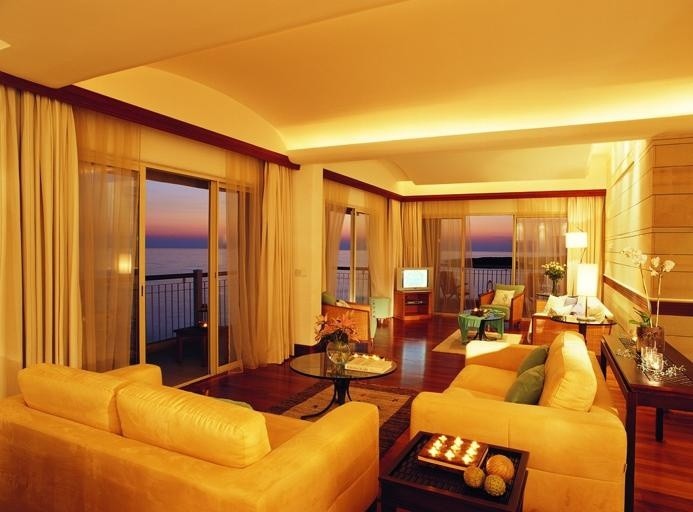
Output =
[
  {"x1": 565, "y1": 232, "x2": 599, "y2": 297},
  {"x1": 114, "y1": 254, "x2": 132, "y2": 274},
  {"x1": 577, "y1": 262, "x2": 598, "y2": 321}
]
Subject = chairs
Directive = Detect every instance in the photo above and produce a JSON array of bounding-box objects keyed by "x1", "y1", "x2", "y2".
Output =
[
  {"x1": 479, "y1": 284, "x2": 525, "y2": 330},
  {"x1": 440, "y1": 270, "x2": 470, "y2": 310},
  {"x1": 322, "y1": 293, "x2": 377, "y2": 342}
]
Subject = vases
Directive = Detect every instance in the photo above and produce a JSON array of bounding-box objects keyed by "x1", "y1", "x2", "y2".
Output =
[
  {"x1": 636, "y1": 325, "x2": 665, "y2": 354},
  {"x1": 551, "y1": 279, "x2": 559, "y2": 296},
  {"x1": 326, "y1": 341, "x2": 350, "y2": 365}
]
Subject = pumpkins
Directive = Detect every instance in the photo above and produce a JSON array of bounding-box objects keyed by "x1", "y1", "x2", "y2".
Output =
[{"x1": 486, "y1": 454, "x2": 515, "y2": 482}]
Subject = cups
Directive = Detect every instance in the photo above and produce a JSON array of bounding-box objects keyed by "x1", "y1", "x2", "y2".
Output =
[{"x1": 641, "y1": 347, "x2": 664, "y2": 372}]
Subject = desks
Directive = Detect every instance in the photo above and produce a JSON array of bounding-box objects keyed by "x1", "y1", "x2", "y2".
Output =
[
  {"x1": 172, "y1": 325, "x2": 225, "y2": 367},
  {"x1": 600, "y1": 334, "x2": 693, "y2": 512},
  {"x1": 458, "y1": 309, "x2": 506, "y2": 344},
  {"x1": 378, "y1": 431, "x2": 531, "y2": 512},
  {"x1": 289, "y1": 351, "x2": 398, "y2": 420}
]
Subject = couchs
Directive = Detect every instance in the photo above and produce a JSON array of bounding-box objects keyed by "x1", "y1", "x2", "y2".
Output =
[
  {"x1": 527, "y1": 294, "x2": 617, "y2": 357},
  {"x1": 0, "y1": 363, "x2": 380, "y2": 512},
  {"x1": 408, "y1": 339, "x2": 627, "y2": 512}
]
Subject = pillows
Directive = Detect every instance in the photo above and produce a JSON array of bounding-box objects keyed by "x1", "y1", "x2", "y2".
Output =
[
  {"x1": 491, "y1": 290, "x2": 515, "y2": 308},
  {"x1": 215, "y1": 397, "x2": 253, "y2": 410},
  {"x1": 543, "y1": 294, "x2": 600, "y2": 319},
  {"x1": 506, "y1": 345, "x2": 547, "y2": 404},
  {"x1": 336, "y1": 300, "x2": 351, "y2": 308}
]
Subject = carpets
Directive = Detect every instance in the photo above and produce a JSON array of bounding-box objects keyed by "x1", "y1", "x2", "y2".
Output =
[
  {"x1": 432, "y1": 328, "x2": 523, "y2": 356},
  {"x1": 264, "y1": 380, "x2": 419, "y2": 460}
]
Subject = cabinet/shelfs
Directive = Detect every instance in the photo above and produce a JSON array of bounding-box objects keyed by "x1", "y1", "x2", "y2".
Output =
[{"x1": 394, "y1": 290, "x2": 434, "y2": 320}]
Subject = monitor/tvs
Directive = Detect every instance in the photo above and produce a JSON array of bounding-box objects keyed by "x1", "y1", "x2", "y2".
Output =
[{"x1": 397, "y1": 266, "x2": 435, "y2": 292}]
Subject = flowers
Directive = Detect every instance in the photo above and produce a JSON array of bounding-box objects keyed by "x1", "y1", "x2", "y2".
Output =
[
  {"x1": 313, "y1": 309, "x2": 362, "y2": 357},
  {"x1": 628, "y1": 254, "x2": 676, "y2": 328},
  {"x1": 541, "y1": 261, "x2": 567, "y2": 280}
]
